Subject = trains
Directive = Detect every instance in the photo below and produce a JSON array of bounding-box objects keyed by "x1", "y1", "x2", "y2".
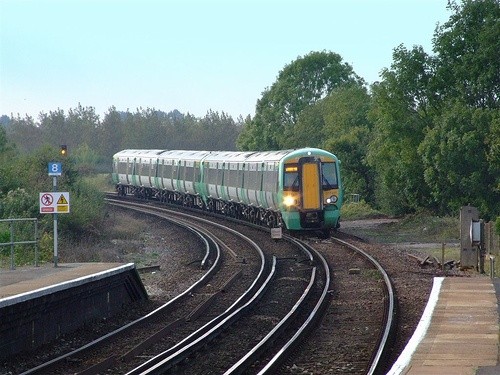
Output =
[{"x1": 111, "y1": 149, "x2": 343, "y2": 237}]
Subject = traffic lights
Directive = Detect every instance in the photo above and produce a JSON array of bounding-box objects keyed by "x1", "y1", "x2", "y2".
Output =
[{"x1": 60, "y1": 143, "x2": 68, "y2": 157}]
